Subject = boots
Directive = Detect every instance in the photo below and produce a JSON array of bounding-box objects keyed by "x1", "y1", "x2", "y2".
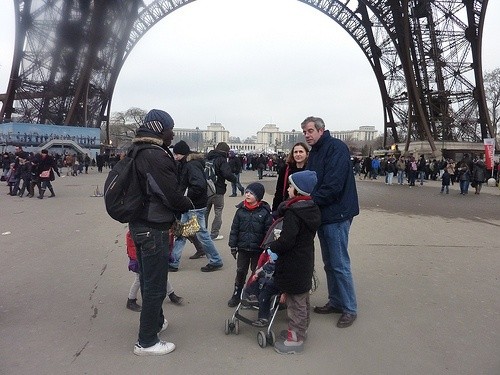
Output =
[
  {"x1": 126, "y1": 297, "x2": 142, "y2": 312},
  {"x1": 168, "y1": 292, "x2": 185, "y2": 306}
]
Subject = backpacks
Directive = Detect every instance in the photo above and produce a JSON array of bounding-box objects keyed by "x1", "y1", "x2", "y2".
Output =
[
  {"x1": 103, "y1": 143, "x2": 171, "y2": 223},
  {"x1": 191, "y1": 160, "x2": 218, "y2": 199}
]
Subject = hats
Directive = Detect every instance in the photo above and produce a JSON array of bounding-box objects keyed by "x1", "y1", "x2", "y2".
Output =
[
  {"x1": 173, "y1": 140, "x2": 190, "y2": 155},
  {"x1": 245, "y1": 182, "x2": 265, "y2": 201},
  {"x1": 289, "y1": 170, "x2": 317, "y2": 197},
  {"x1": 139, "y1": 109, "x2": 175, "y2": 135},
  {"x1": 216, "y1": 142, "x2": 230, "y2": 154}
]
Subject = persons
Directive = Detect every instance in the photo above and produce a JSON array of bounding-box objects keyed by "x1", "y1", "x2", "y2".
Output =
[
  {"x1": 128, "y1": 109, "x2": 195, "y2": 355},
  {"x1": 0, "y1": 140, "x2": 500, "y2": 326},
  {"x1": 301, "y1": 117, "x2": 359, "y2": 329}
]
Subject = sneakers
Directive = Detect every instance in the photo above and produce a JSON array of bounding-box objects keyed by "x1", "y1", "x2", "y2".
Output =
[
  {"x1": 133, "y1": 340, "x2": 176, "y2": 356},
  {"x1": 157, "y1": 318, "x2": 168, "y2": 337}
]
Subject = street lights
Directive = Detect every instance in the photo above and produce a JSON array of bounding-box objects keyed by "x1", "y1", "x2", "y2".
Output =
[
  {"x1": 195, "y1": 126, "x2": 200, "y2": 154},
  {"x1": 292, "y1": 128, "x2": 295, "y2": 147},
  {"x1": 275, "y1": 138, "x2": 278, "y2": 155}
]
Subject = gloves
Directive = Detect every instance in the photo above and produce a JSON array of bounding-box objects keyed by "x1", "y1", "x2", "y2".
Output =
[{"x1": 230, "y1": 247, "x2": 239, "y2": 261}]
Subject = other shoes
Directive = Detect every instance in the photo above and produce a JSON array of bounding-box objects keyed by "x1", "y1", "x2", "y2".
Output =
[
  {"x1": 337, "y1": 312, "x2": 357, "y2": 328},
  {"x1": 314, "y1": 303, "x2": 332, "y2": 314},
  {"x1": 201, "y1": 262, "x2": 224, "y2": 272},
  {"x1": 190, "y1": 250, "x2": 205, "y2": 259},
  {"x1": 228, "y1": 297, "x2": 240, "y2": 307},
  {"x1": 4, "y1": 191, "x2": 55, "y2": 199},
  {"x1": 212, "y1": 235, "x2": 224, "y2": 240},
  {"x1": 240, "y1": 187, "x2": 244, "y2": 196},
  {"x1": 229, "y1": 193, "x2": 237, "y2": 197},
  {"x1": 273, "y1": 329, "x2": 308, "y2": 355}
]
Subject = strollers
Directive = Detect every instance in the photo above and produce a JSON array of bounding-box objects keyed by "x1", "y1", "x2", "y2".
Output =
[{"x1": 225, "y1": 218, "x2": 318, "y2": 349}]
